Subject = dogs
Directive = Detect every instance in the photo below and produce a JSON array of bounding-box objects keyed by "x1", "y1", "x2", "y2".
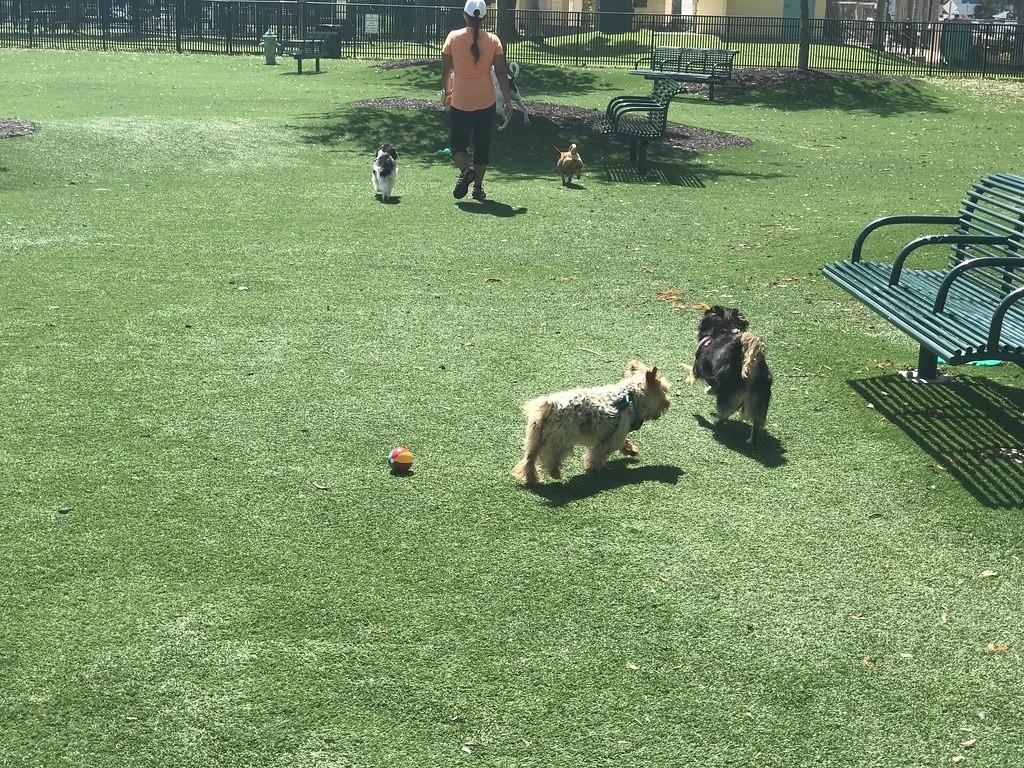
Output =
[
  {"x1": 556, "y1": 142, "x2": 584, "y2": 186},
  {"x1": 685, "y1": 304, "x2": 773, "y2": 446},
  {"x1": 439, "y1": 61, "x2": 530, "y2": 133},
  {"x1": 370, "y1": 142, "x2": 398, "y2": 201},
  {"x1": 511, "y1": 358, "x2": 672, "y2": 485}
]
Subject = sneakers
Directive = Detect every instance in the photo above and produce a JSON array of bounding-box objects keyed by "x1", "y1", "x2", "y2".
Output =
[
  {"x1": 453, "y1": 167, "x2": 476, "y2": 199},
  {"x1": 472, "y1": 185, "x2": 486, "y2": 199}
]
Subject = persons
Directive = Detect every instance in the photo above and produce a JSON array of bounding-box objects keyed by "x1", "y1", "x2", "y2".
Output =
[{"x1": 441, "y1": 0, "x2": 512, "y2": 199}]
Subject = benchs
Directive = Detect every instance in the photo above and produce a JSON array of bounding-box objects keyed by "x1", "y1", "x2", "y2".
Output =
[
  {"x1": 629, "y1": 46, "x2": 740, "y2": 101},
  {"x1": 819, "y1": 173, "x2": 1024, "y2": 385},
  {"x1": 592, "y1": 77, "x2": 687, "y2": 177},
  {"x1": 289, "y1": 32, "x2": 328, "y2": 74}
]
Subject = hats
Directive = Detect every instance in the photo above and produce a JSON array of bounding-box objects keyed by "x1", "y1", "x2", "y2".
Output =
[{"x1": 464, "y1": 0, "x2": 487, "y2": 18}]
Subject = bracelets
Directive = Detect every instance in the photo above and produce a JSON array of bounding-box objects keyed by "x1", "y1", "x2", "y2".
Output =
[{"x1": 445, "y1": 92, "x2": 451, "y2": 95}]
